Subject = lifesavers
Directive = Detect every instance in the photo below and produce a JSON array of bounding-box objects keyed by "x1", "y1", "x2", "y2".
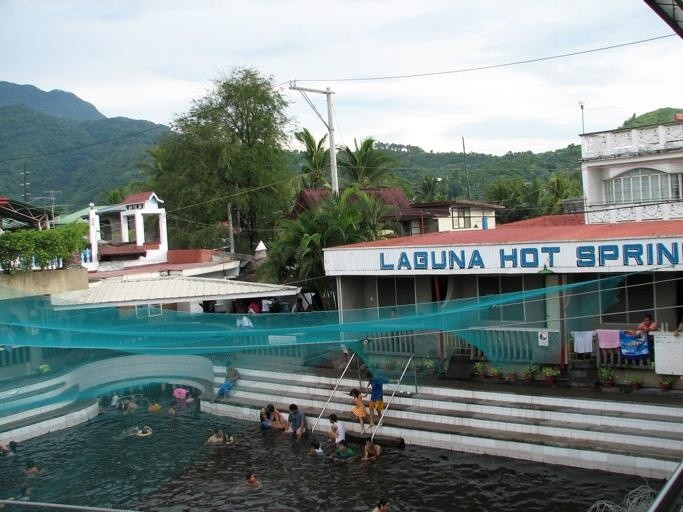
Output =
[{"x1": 136, "y1": 430, "x2": 152, "y2": 436}]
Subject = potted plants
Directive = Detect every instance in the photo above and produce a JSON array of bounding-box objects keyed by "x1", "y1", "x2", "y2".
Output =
[{"x1": 384, "y1": 357, "x2": 675, "y2": 392}]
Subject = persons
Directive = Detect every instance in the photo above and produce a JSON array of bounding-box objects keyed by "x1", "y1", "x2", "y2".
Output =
[
  {"x1": 38, "y1": 361, "x2": 51, "y2": 375},
  {"x1": 22, "y1": 460, "x2": 39, "y2": 474},
  {"x1": 184, "y1": 390, "x2": 195, "y2": 402},
  {"x1": 20, "y1": 485, "x2": 34, "y2": 497},
  {"x1": 109, "y1": 387, "x2": 175, "y2": 416},
  {"x1": 634, "y1": 315, "x2": 659, "y2": 366},
  {"x1": 235, "y1": 297, "x2": 303, "y2": 328},
  {"x1": 210, "y1": 361, "x2": 241, "y2": 403},
  {"x1": 195, "y1": 427, "x2": 223, "y2": 452},
  {"x1": 253, "y1": 398, "x2": 382, "y2": 473},
  {"x1": 137, "y1": 423, "x2": 152, "y2": 434},
  {"x1": 233, "y1": 471, "x2": 263, "y2": 494},
  {"x1": 222, "y1": 433, "x2": 234, "y2": 444},
  {"x1": 346, "y1": 388, "x2": 369, "y2": 434},
  {"x1": 674, "y1": 323, "x2": 683, "y2": 336},
  {"x1": 0, "y1": 441, "x2": 17, "y2": 460},
  {"x1": 365, "y1": 367, "x2": 390, "y2": 430},
  {"x1": 371, "y1": 496, "x2": 391, "y2": 512}
]
